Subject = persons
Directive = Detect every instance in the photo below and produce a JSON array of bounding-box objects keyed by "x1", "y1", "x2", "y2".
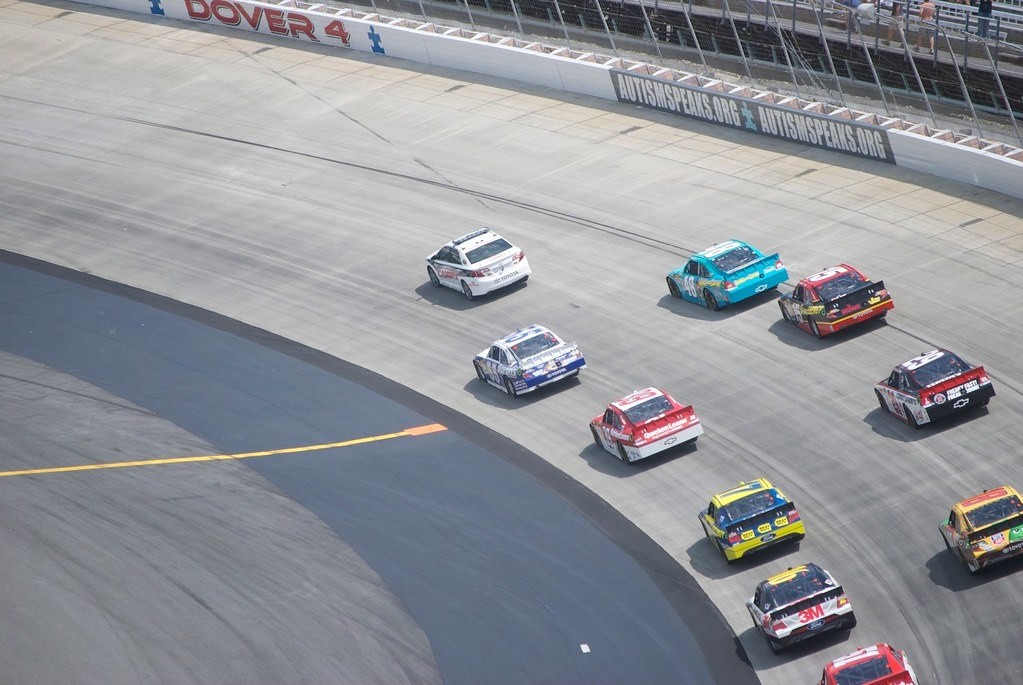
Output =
[
  {"x1": 880, "y1": 0, "x2": 907, "y2": 47},
  {"x1": 913, "y1": 0, "x2": 935, "y2": 55},
  {"x1": 974, "y1": 0, "x2": 993, "y2": 37}
]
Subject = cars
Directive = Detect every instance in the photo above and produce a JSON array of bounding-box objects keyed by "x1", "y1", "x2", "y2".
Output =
[
  {"x1": 423, "y1": 226, "x2": 531, "y2": 302},
  {"x1": 818, "y1": 642, "x2": 919, "y2": 684},
  {"x1": 666, "y1": 239, "x2": 790, "y2": 312},
  {"x1": 778, "y1": 264, "x2": 895, "y2": 339},
  {"x1": 588, "y1": 386, "x2": 705, "y2": 465},
  {"x1": 744, "y1": 561, "x2": 859, "y2": 656},
  {"x1": 937, "y1": 481, "x2": 1023, "y2": 575},
  {"x1": 872, "y1": 348, "x2": 995, "y2": 430},
  {"x1": 473, "y1": 324, "x2": 590, "y2": 399},
  {"x1": 698, "y1": 476, "x2": 807, "y2": 568}
]
